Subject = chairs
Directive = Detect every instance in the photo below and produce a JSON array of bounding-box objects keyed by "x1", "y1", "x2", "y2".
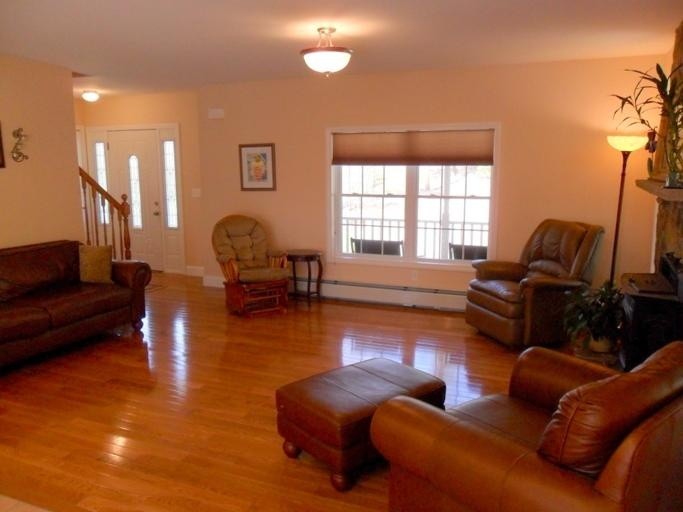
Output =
[
  {"x1": 465, "y1": 218, "x2": 605, "y2": 350},
  {"x1": 369, "y1": 339, "x2": 683, "y2": 511},
  {"x1": 211, "y1": 214, "x2": 291, "y2": 319}
]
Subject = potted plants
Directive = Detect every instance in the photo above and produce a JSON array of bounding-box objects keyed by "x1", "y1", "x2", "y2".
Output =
[
  {"x1": 560, "y1": 279, "x2": 634, "y2": 353},
  {"x1": 610, "y1": 59, "x2": 683, "y2": 187}
]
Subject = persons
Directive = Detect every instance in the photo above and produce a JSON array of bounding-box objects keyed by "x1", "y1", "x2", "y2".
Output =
[{"x1": 249, "y1": 153, "x2": 266, "y2": 182}]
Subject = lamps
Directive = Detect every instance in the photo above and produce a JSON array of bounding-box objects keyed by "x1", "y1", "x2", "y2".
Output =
[
  {"x1": 607, "y1": 135, "x2": 648, "y2": 289},
  {"x1": 297, "y1": 26, "x2": 357, "y2": 77}
]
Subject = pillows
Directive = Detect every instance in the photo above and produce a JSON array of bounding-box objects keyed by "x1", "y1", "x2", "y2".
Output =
[{"x1": 77, "y1": 243, "x2": 114, "y2": 285}]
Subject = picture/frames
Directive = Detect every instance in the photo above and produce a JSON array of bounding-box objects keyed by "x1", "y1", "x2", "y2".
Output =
[{"x1": 237, "y1": 143, "x2": 276, "y2": 192}]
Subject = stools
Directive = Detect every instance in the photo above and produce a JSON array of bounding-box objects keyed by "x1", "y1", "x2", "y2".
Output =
[
  {"x1": 286, "y1": 248, "x2": 324, "y2": 301},
  {"x1": 275, "y1": 356, "x2": 446, "y2": 493}
]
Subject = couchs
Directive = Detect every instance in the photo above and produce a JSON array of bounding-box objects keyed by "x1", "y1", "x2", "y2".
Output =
[{"x1": 0, "y1": 240, "x2": 152, "y2": 364}]
